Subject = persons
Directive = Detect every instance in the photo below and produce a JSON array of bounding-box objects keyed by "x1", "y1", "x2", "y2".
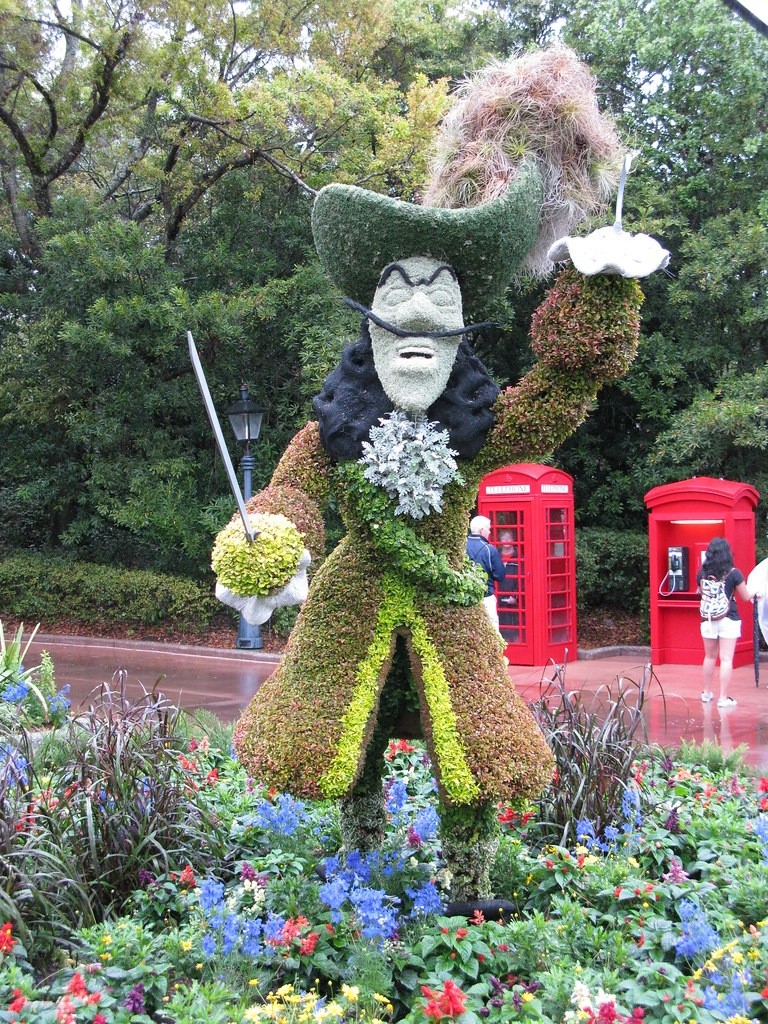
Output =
[
  {"x1": 212, "y1": 45, "x2": 670, "y2": 921},
  {"x1": 496, "y1": 525, "x2": 520, "y2": 635},
  {"x1": 465, "y1": 515, "x2": 509, "y2": 631},
  {"x1": 695, "y1": 538, "x2": 758, "y2": 708}
]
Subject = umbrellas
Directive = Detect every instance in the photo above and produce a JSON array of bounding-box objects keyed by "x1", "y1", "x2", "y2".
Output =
[{"x1": 753, "y1": 593, "x2": 760, "y2": 687}]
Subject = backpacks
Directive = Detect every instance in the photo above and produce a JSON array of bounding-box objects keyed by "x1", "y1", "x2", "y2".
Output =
[{"x1": 700, "y1": 567, "x2": 736, "y2": 620}]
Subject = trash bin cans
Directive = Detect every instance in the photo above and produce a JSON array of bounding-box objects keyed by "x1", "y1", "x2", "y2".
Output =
[{"x1": 236, "y1": 612, "x2": 265, "y2": 652}]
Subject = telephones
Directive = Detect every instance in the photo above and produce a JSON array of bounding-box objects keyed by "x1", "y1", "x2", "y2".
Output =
[{"x1": 668, "y1": 546, "x2": 690, "y2": 590}]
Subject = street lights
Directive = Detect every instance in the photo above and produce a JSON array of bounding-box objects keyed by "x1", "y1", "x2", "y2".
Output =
[{"x1": 226, "y1": 382, "x2": 269, "y2": 651}]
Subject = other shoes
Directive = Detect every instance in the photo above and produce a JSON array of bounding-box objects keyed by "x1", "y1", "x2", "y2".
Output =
[
  {"x1": 701, "y1": 691, "x2": 713, "y2": 701},
  {"x1": 717, "y1": 697, "x2": 737, "y2": 707}
]
